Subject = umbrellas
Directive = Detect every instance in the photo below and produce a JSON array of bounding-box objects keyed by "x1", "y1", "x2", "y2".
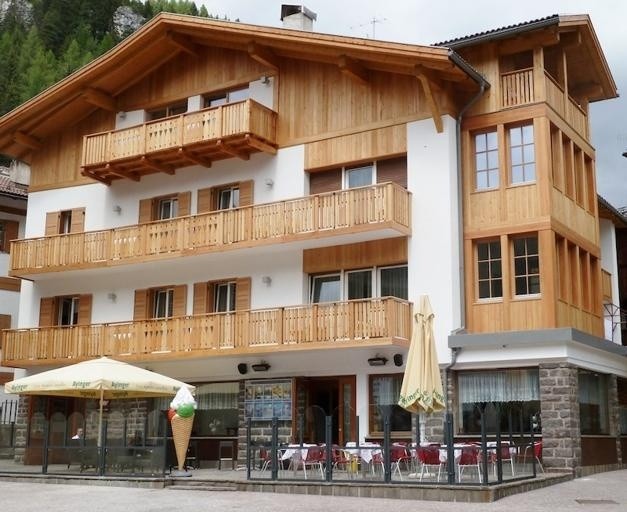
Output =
[
  {"x1": 4, "y1": 356, "x2": 197, "y2": 448},
  {"x1": 399, "y1": 294, "x2": 447, "y2": 451}
]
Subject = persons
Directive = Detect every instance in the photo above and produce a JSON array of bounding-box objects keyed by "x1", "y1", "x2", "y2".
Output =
[
  {"x1": 120, "y1": 428, "x2": 144, "y2": 468},
  {"x1": 70, "y1": 427, "x2": 88, "y2": 472}
]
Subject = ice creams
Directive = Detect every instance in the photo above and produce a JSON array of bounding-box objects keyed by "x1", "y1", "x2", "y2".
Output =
[{"x1": 168, "y1": 386, "x2": 198, "y2": 471}]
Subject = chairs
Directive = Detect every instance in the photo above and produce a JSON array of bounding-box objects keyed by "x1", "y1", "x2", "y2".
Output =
[
  {"x1": 260, "y1": 441, "x2": 544, "y2": 484},
  {"x1": 67, "y1": 439, "x2": 200, "y2": 474}
]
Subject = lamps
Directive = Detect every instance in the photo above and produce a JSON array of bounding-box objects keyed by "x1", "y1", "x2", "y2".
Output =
[
  {"x1": 368, "y1": 357, "x2": 387, "y2": 366},
  {"x1": 261, "y1": 76, "x2": 270, "y2": 84},
  {"x1": 262, "y1": 277, "x2": 271, "y2": 283},
  {"x1": 118, "y1": 111, "x2": 126, "y2": 118},
  {"x1": 252, "y1": 364, "x2": 270, "y2": 371},
  {"x1": 108, "y1": 293, "x2": 117, "y2": 299},
  {"x1": 113, "y1": 206, "x2": 121, "y2": 212},
  {"x1": 265, "y1": 179, "x2": 274, "y2": 185}
]
